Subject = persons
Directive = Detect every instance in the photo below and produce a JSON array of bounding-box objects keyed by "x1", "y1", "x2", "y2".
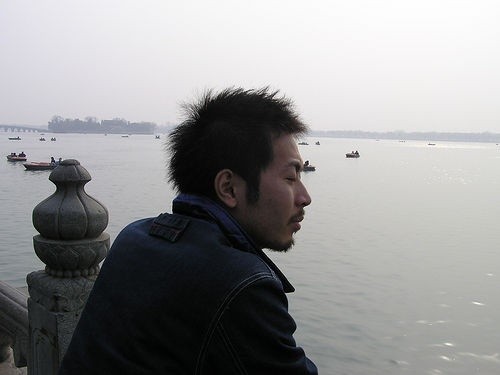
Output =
[
  {"x1": 303, "y1": 160, "x2": 310, "y2": 166},
  {"x1": 12, "y1": 136, "x2": 62, "y2": 163},
  {"x1": 58, "y1": 85, "x2": 319, "y2": 375},
  {"x1": 352, "y1": 150, "x2": 359, "y2": 155}
]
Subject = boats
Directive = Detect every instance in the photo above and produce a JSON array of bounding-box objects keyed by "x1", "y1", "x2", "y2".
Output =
[
  {"x1": 122, "y1": 136, "x2": 128, "y2": 137},
  {"x1": 8, "y1": 137, "x2": 21, "y2": 140},
  {"x1": 7, "y1": 152, "x2": 27, "y2": 161},
  {"x1": 23, "y1": 161, "x2": 63, "y2": 169},
  {"x1": 40, "y1": 139, "x2": 45, "y2": 141},
  {"x1": 51, "y1": 139, "x2": 56, "y2": 141},
  {"x1": 155, "y1": 137, "x2": 160, "y2": 139}
]
